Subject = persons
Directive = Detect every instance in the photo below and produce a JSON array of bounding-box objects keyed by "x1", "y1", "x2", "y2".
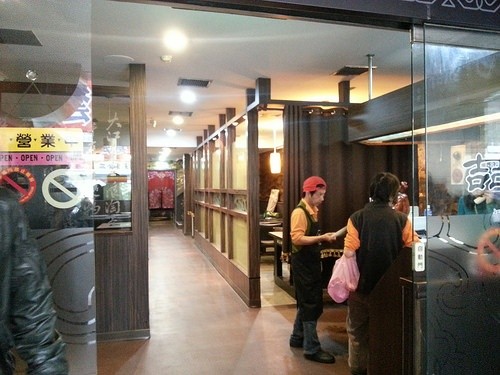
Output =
[
  {"x1": 393, "y1": 182, "x2": 410, "y2": 217},
  {"x1": 0, "y1": 187, "x2": 69, "y2": 375},
  {"x1": 54, "y1": 200, "x2": 93, "y2": 230},
  {"x1": 289, "y1": 176, "x2": 336, "y2": 364},
  {"x1": 344, "y1": 172, "x2": 423, "y2": 375},
  {"x1": 458, "y1": 172, "x2": 498, "y2": 216}
]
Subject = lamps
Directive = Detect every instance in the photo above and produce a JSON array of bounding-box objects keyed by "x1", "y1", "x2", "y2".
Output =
[{"x1": 270, "y1": 126, "x2": 281, "y2": 174}]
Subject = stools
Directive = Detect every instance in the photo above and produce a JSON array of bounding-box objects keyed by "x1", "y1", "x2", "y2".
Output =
[{"x1": 261, "y1": 240, "x2": 274, "y2": 256}]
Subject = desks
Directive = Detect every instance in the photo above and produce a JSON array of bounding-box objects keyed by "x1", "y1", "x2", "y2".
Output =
[{"x1": 260, "y1": 219, "x2": 281, "y2": 256}]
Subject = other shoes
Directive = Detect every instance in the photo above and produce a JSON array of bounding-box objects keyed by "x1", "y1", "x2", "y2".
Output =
[
  {"x1": 289, "y1": 339, "x2": 304, "y2": 348},
  {"x1": 304, "y1": 349, "x2": 335, "y2": 363}
]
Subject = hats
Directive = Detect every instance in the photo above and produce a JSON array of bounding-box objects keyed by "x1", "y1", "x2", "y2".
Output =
[{"x1": 302, "y1": 176, "x2": 327, "y2": 192}]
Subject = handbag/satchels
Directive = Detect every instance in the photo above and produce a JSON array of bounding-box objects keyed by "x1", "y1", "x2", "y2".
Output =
[{"x1": 327, "y1": 251, "x2": 360, "y2": 304}]
